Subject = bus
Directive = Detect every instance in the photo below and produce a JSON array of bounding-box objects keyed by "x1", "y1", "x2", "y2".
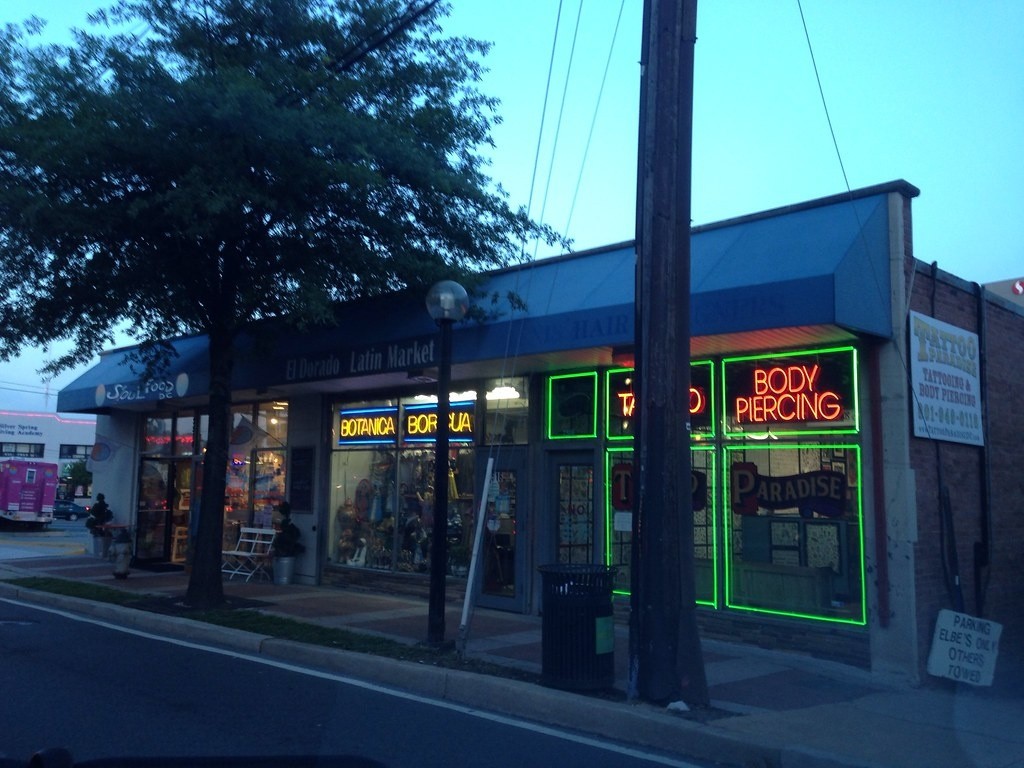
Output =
[{"x1": 1, "y1": 456, "x2": 58, "y2": 530}]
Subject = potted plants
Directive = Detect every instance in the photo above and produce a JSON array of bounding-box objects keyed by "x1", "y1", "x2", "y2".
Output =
[
  {"x1": 85, "y1": 492, "x2": 114, "y2": 561},
  {"x1": 271, "y1": 501, "x2": 307, "y2": 584}
]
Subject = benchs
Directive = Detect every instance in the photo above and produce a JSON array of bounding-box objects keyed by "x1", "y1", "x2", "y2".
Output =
[{"x1": 221, "y1": 527, "x2": 279, "y2": 582}]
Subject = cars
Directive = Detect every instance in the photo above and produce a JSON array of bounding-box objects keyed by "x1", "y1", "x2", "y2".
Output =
[{"x1": 53, "y1": 499, "x2": 91, "y2": 522}]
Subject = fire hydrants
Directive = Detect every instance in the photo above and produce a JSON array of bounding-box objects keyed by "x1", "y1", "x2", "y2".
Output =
[{"x1": 109, "y1": 527, "x2": 136, "y2": 578}]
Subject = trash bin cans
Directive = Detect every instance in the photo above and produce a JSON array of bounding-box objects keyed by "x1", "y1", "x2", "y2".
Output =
[{"x1": 536, "y1": 562, "x2": 618, "y2": 692}]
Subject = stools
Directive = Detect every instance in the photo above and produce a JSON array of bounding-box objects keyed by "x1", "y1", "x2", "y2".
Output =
[{"x1": 170, "y1": 522, "x2": 189, "y2": 562}]
